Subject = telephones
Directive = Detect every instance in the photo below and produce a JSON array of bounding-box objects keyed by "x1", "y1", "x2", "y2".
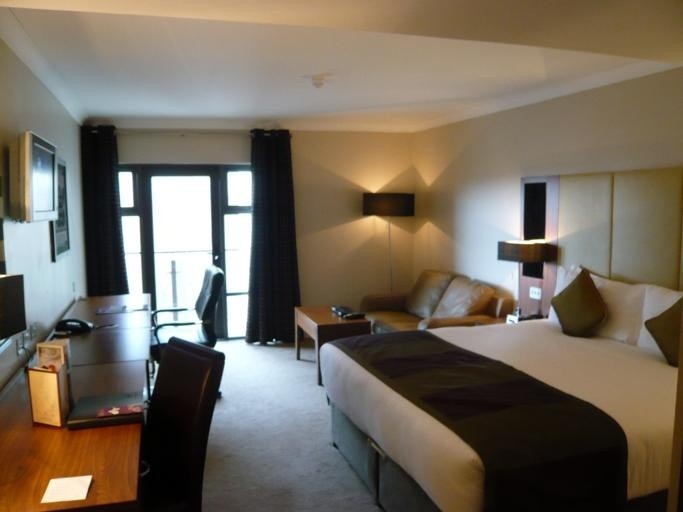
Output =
[{"x1": 56, "y1": 318, "x2": 94, "y2": 333}]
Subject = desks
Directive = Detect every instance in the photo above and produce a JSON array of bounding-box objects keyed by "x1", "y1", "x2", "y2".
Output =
[{"x1": 0, "y1": 294, "x2": 151, "y2": 512}]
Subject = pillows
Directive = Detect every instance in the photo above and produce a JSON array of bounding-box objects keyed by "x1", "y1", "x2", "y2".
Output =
[
  {"x1": 551, "y1": 266, "x2": 683, "y2": 367},
  {"x1": 406, "y1": 268, "x2": 454, "y2": 319},
  {"x1": 432, "y1": 276, "x2": 495, "y2": 320}
]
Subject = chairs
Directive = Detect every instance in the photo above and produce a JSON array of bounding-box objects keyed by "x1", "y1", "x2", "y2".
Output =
[
  {"x1": 152, "y1": 265, "x2": 224, "y2": 399},
  {"x1": 141, "y1": 336, "x2": 225, "y2": 512}
]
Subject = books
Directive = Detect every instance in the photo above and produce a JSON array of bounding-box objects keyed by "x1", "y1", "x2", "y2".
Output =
[
  {"x1": 36, "y1": 338, "x2": 75, "y2": 412},
  {"x1": 26, "y1": 361, "x2": 70, "y2": 429},
  {"x1": 66, "y1": 390, "x2": 145, "y2": 431}
]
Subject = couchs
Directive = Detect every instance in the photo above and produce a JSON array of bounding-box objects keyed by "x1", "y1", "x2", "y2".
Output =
[{"x1": 362, "y1": 271, "x2": 513, "y2": 333}]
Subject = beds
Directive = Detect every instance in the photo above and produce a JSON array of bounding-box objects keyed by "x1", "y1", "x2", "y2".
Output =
[{"x1": 318, "y1": 268, "x2": 683, "y2": 511}]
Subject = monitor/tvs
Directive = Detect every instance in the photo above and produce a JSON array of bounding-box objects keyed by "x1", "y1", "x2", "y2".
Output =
[{"x1": 7, "y1": 129, "x2": 59, "y2": 223}]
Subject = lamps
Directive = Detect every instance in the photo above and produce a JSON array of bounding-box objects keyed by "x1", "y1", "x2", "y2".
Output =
[
  {"x1": 498, "y1": 240, "x2": 558, "y2": 264},
  {"x1": 363, "y1": 193, "x2": 415, "y2": 292}
]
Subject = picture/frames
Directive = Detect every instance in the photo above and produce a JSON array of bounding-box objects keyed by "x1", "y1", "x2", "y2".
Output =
[{"x1": 51, "y1": 160, "x2": 71, "y2": 262}]
